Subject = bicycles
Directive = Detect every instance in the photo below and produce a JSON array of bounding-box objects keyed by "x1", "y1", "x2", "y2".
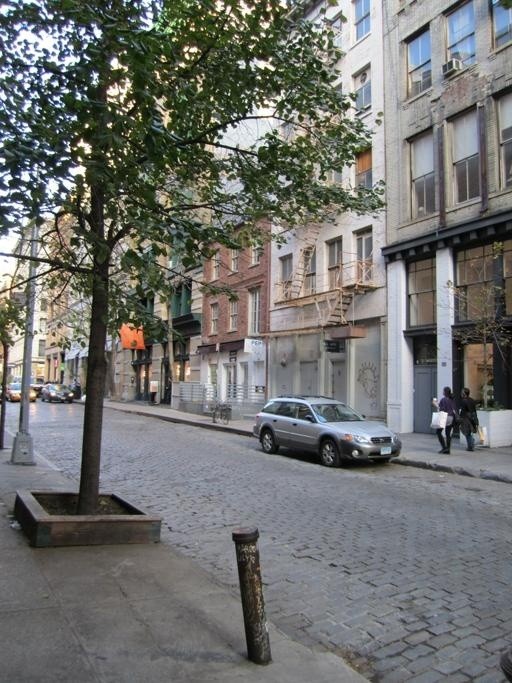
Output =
[{"x1": 210, "y1": 396, "x2": 232, "y2": 425}]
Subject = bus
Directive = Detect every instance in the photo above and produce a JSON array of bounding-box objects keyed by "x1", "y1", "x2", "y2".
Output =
[{"x1": 14, "y1": 376, "x2": 44, "y2": 395}]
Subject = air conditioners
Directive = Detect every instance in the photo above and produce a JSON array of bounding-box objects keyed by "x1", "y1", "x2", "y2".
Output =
[{"x1": 440, "y1": 58, "x2": 464, "y2": 76}]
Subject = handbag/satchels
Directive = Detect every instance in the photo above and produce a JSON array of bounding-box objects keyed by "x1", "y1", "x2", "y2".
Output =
[
  {"x1": 471, "y1": 411, "x2": 479, "y2": 425},
  {"x1": 430, "y1": 411, "x2": 448, "y2": 429},
  {"x1": 455, "y1": 413, "x2": 464, "y2": 423}
]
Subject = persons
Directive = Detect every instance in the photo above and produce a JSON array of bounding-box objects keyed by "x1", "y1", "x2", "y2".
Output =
[
  {"x1": 457, "y1": 388, "x2": 479, "y2": 451},
  {"x1": 432, "y1": 386, "x2": 458, "y2": 455}
]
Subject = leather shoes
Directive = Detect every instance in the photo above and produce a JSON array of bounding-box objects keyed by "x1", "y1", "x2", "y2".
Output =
[{"x1": 439, "y1": 444, "x2": 475, "y2": 454}]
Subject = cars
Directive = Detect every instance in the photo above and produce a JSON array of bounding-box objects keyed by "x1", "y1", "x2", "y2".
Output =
[
  {"x1": 252, "y1": 394, "x2": 401, "y2": 468},
  {"x1": 41, "y1": 383, "x2": 74, "y2": 403},
  {"x1": 6, "y1": 383, "x2": 37, "y2": 402}
]
